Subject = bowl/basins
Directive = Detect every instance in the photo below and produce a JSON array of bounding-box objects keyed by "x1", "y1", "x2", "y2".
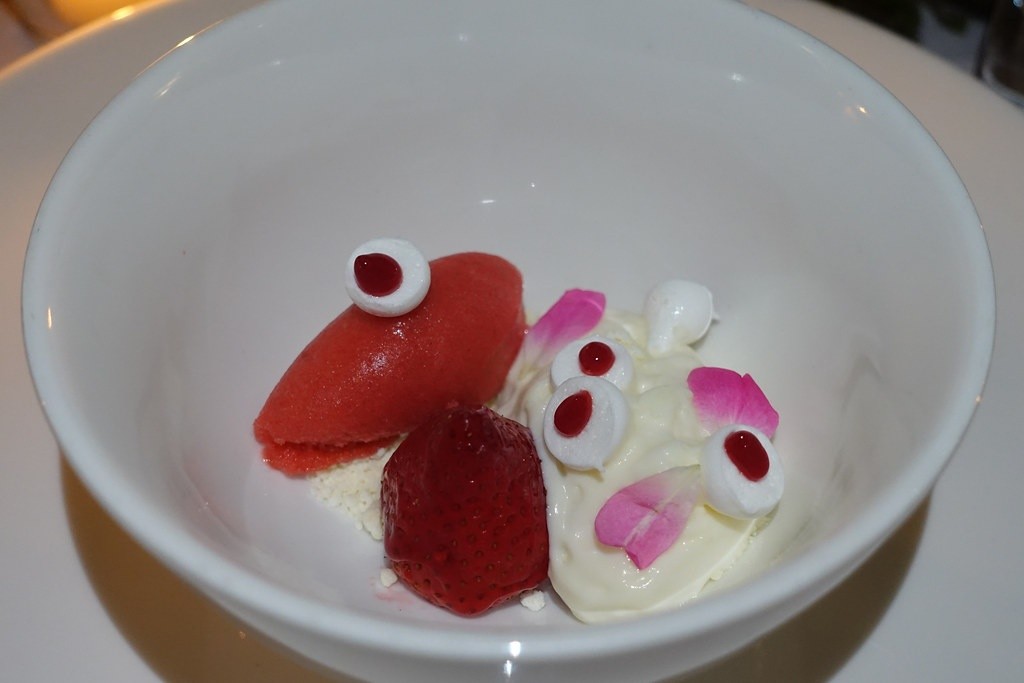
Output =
[{"x1": 22, "y1": 0, "x2": 997, "y2": 682}]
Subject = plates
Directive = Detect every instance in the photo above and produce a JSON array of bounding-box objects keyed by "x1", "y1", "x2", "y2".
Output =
[{"x1": 0, "y1": 0, "x2": 1024, "y2": 683}]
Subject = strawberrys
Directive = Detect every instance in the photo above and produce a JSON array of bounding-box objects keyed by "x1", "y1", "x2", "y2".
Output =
[{"x1": 379, "y1": 401, "x2": 550, "y2": 616}]
